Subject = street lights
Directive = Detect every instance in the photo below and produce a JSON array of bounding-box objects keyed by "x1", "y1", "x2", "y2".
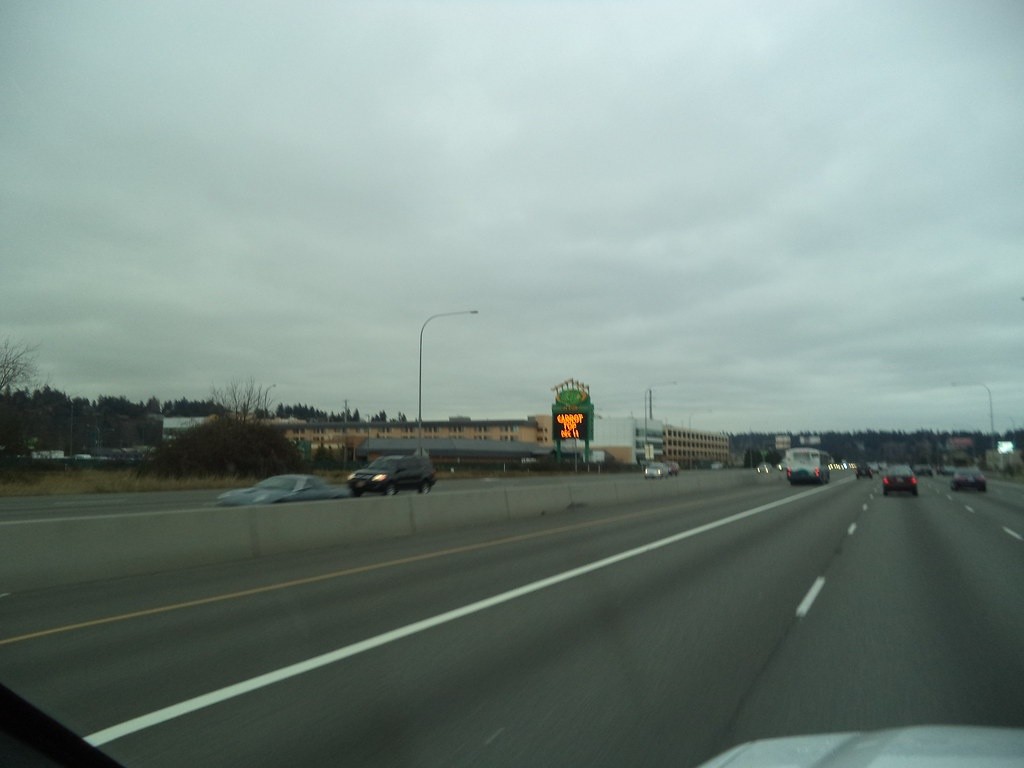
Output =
[
  {"x1": 952, "y1": 383, "x2": 995, "y2": 475},
  {"x1": 263, "y1": 385, "x2": 276, "y2": 417},
  {"x1": 643, "y1": 382, "x2": 677, "y2": 467},
  {"x1": 418, "y1": 310, "x2": 480, "y2": 457}
]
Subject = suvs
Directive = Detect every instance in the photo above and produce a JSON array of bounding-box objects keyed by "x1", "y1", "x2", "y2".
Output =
[
  {"x1": 348, "y1": 455, "x2": 439, "y2": 495},
  {"x1": 882, "y1": 465, "x2": 918, "y2": 497},
  {"x1": 855, "y1": 465, "x2": 872, "y2": 479}
]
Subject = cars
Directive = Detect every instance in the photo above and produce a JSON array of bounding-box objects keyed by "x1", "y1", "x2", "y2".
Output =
[
  {"x1": 756, "y1": 462, "x2": 772, "y2": 474},
  {"x1": 216, "y1": 475, "x2": 352, "y2": 506},
  {"x1": 870, "y1": 465, "x2": 879, "y2": 474},
  {"x1": 645, "y1": 462, "x2": 680, "y2": 478},
  {"x1": 950, "y1": 467, "x2": 986, "y2": 492},
  {"x1": 711, "y1": 461, "x2": 723, "y2": 470},
  {"x1": 939, "y1": 466, "x2": 953, "y2": 476},
  {"x1": 913, "y1": 464, "x2": 932, "y2": 476}
]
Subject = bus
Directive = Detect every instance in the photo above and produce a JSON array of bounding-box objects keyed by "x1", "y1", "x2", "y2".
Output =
[{"x1": 786, "y1": 448, "x2": 831, "y2": 485}]
